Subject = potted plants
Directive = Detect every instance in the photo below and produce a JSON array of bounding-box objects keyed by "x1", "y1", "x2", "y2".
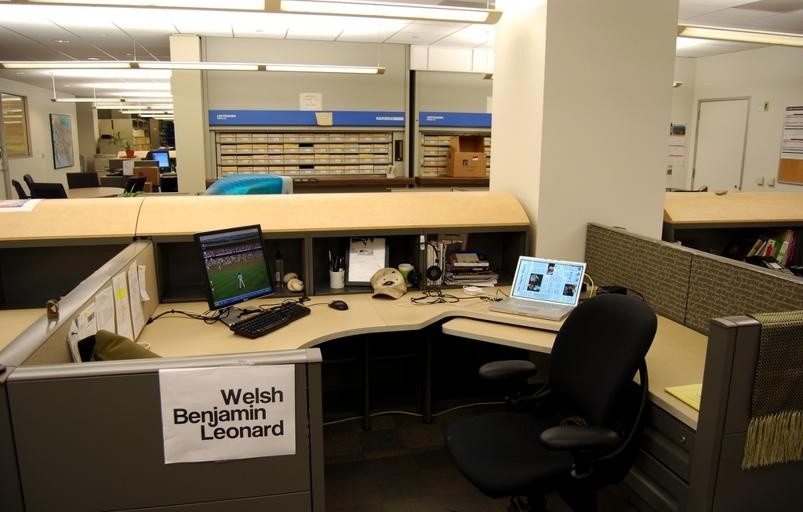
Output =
[{"x1": 124, "y1": 141, "x2": 135, "y2": 157}]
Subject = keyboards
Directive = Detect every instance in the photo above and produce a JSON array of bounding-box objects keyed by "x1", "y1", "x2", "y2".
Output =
[{"x1": 228, "y1": 301, "x2": 311, "y2": 340}]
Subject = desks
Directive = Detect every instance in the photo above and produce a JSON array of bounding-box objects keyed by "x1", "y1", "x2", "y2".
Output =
[
  {"x1": 0, "y1": 308, "x2": 52, "y2": 351},
  {"x1": 137, "y1": 285, "x2": 712, "y2": 511}
]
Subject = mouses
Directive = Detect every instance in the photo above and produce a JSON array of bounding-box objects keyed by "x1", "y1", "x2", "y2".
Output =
[{"x1": 328, "y1": 299, "x2": 348, "y2": 310}]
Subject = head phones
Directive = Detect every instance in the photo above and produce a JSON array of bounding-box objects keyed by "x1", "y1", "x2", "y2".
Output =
[{"x1": 407, "y1": 242, "x2": 442, "y2": 285}]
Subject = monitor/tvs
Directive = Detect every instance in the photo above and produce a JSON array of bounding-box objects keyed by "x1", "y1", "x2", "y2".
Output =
[
  {"x1": 192, "y1": 224, "x2": 278, "y2": 327},
  {"x1": 146, "y1": 150, "x2": 171, "y2": 173},
  {"x1": 203, "y1": 173, "x2": 293, "y2": 196}
]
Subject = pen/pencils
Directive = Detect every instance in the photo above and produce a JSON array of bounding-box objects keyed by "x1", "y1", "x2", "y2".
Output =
[{"x1": 328, "y1": 248, "x2": 345, "y2": 272}]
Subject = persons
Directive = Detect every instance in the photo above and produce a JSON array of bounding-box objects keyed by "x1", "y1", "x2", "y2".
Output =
[{"x1": 237, "y1": 272, "x2": 246, "y2": 288}]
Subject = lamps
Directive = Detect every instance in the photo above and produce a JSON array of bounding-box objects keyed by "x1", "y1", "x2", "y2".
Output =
[
  {"x1": 265, "y1": 0, "x2": 503, "y2": 25},
  {"x1": 50, "y1": 75, "x2": 174, "y2": 121},
  {"x1": 677, "y1": 25, "x2": 803, "y2": 48},
  {"x1": 0, "y1": 29, "x2": 386, "y2": 74}
]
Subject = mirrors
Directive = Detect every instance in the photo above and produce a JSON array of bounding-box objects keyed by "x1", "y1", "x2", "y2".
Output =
[{"x1": 0, "y1": 92, "x2": 32, "y2": 159}]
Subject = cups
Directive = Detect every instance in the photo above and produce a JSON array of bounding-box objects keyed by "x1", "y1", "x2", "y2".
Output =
[
  {"x1": 329, "y1": 270, "x2": 344, "y2": 289},
  {"x1": 398, "y1": 263, "x2": 415, "y2": 288}
]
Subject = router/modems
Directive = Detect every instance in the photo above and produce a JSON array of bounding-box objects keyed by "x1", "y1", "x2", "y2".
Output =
[{"x1": 579, "y1": 281, "x2": 599, "y2": 299}]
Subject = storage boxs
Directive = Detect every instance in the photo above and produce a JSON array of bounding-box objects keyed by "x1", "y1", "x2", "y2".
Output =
[{"x1": 447, "y1": 134, "x2": 486, "y2": 178}]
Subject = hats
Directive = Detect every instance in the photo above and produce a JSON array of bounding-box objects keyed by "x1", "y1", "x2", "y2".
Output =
[{"x1": 372, "y1": 268, "x2": 407, "y2": 299}]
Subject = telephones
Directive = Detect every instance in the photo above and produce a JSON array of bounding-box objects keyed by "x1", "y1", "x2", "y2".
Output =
[{"x1": 745, "y1": 255, "x2": 795, "y2": 276}]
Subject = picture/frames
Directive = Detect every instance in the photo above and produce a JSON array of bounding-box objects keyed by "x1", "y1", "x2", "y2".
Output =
[{"x1": 50, "y1": 114, "x2": 74, "y2": 169}]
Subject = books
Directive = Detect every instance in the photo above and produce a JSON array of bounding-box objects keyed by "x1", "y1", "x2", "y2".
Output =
[
  {"x1": 738, "y1": 235, "x2": 789, "y2": 266},
  {"x1": 443, "y1": 252, "x2": 500, "y2": 288}
]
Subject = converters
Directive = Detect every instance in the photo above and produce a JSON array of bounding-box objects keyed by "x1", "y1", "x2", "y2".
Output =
[{"x1": 607, "y1": 285, "x2": 628, "y2": 295}]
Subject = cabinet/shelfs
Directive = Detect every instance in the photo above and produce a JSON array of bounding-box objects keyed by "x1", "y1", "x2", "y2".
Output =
[{"x1": 625, "y1": 399, "x2": 695, "y2": 512}]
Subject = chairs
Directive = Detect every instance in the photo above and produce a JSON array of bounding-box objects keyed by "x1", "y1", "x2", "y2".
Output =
[
  {"x1": 11, "y1": 158, "x2": 160, "y2": 199},
  {"x1": 444, "y1": 293, "x2": 658, "y2": 512}
]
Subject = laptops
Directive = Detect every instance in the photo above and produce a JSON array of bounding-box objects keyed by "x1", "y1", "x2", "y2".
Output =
[{"x1": 487, "y1": 255, "x2": 588, "y2": 322}]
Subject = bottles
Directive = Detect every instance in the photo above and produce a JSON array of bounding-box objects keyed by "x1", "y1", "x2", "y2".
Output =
[
  {"x1": 170, "y1": 160, "x2": 174, "y2": 171},
  {"x1": 275, "y1": 251, "x2": 283, "y2": 287}
]
